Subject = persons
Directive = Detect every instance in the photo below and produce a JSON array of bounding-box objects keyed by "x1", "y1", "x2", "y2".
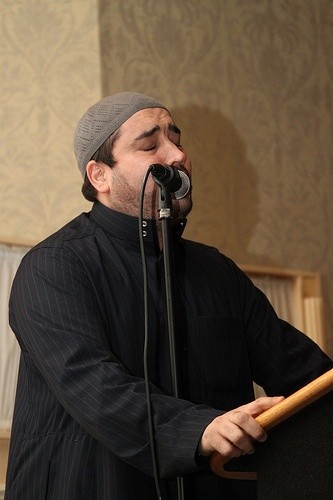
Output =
[{"x1": 4, "y1": 91, "x2": 332, "y2": 499}]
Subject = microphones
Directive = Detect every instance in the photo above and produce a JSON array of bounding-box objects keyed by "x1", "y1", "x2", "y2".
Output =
[{"x1": 149, "y1": 163, "x2": 191, "y2": 200}]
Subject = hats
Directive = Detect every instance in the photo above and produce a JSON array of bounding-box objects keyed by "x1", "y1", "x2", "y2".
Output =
[{"x1": 71, "y1": 92, "x2": 171, "y2": 179}]
